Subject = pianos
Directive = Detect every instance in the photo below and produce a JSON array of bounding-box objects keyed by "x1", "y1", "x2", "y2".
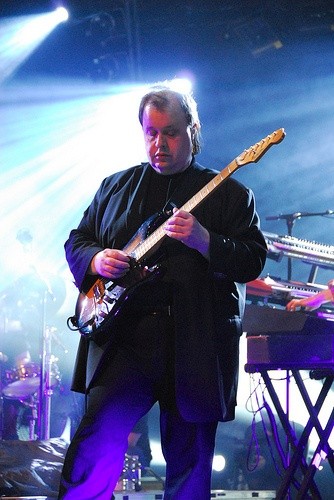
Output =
[{"x1": 239, "y1": 230, "x2": 334, "y2": 500}]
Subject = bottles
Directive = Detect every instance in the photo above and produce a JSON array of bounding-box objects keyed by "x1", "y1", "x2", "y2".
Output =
[{"x1": 122, "y1": 451, "x2": 141, "y2": 490}]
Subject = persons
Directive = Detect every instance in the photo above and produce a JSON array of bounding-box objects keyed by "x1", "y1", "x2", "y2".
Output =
[
  {"x1": 119, "y1": 415, "x2": 153, "y2": 477},
  {"x1": 286, "y1": 279, "x2": 334, "y2": 312},
  {"x1": 56, "y1": 85, "x2": 268, "y2": 500},
  {"x1": 0, "y1": 298, "x2": 47, "y2": 374}
]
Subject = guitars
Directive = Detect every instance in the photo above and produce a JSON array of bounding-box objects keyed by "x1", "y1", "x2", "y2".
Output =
[{"x1": 74, "y1": 127, "x2": 286, "y2": 339}]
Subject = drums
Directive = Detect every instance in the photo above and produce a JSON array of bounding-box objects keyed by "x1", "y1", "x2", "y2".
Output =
[{"x1": 0, "y1": 362, "x2": 61, "y2": 400}]
